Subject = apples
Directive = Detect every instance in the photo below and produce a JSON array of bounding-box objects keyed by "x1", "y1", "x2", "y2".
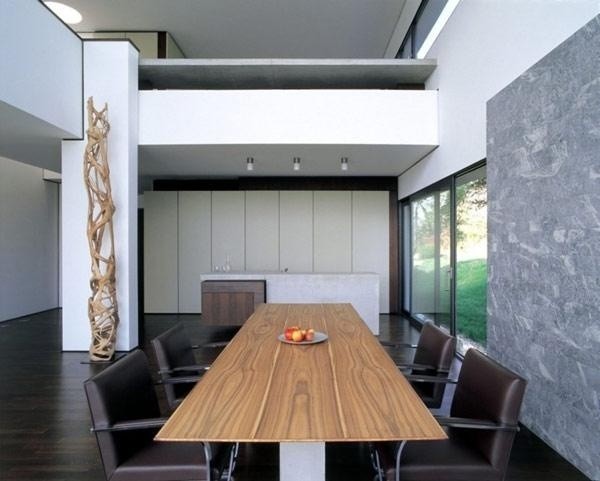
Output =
[{"x1": 285, "y1": 326, "x2": 314, "y2": 342}]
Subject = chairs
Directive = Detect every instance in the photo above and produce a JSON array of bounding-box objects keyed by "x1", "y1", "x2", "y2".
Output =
[
  {"x1": 80, "y1": 319, "x2": 244, "y2": 481},
  {"x1": 367, "y1": 323, "x2": 527, "y2": 481}
]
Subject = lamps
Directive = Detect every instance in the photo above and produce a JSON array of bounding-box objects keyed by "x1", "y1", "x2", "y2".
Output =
[{"x1": 245, "y1": 156, "x2": 351, "y2": 173}]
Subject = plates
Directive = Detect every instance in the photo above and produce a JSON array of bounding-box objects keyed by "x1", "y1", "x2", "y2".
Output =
[{"x1": 277, "y1": 331, "x2": 328, "y2": 346}]
